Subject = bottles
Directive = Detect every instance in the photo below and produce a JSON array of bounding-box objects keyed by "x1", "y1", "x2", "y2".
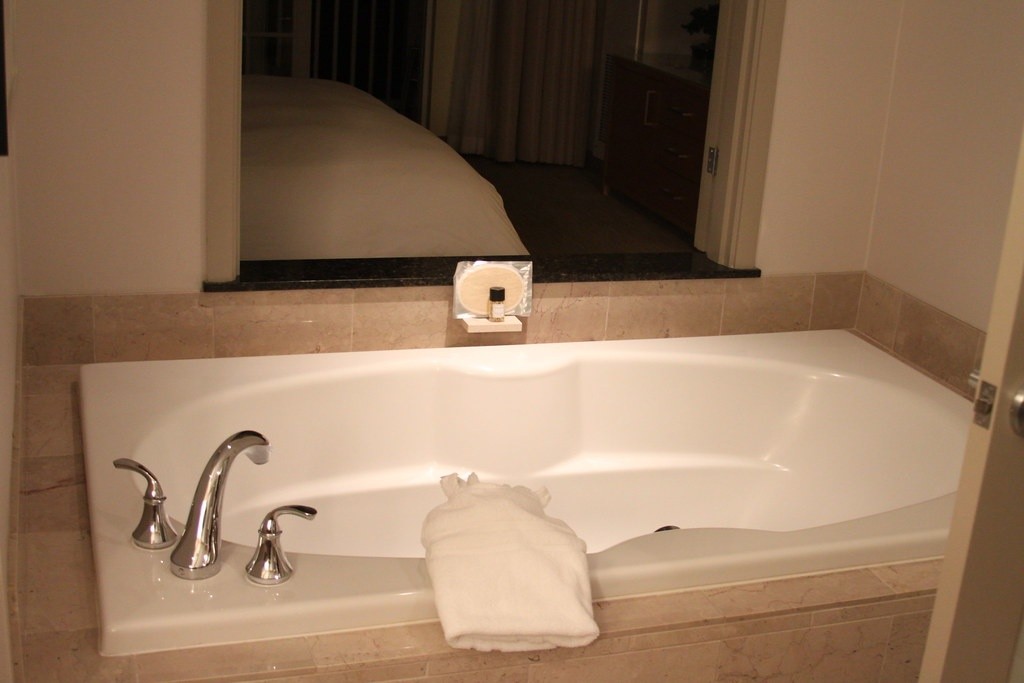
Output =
[{"x1": 488, "y1": 286, "x2": 505, "y2": 322}]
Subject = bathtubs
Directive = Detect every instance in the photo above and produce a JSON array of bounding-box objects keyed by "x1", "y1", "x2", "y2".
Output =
[{"x1": 78, "y1": 328, "x2": 980, "y2": 657}]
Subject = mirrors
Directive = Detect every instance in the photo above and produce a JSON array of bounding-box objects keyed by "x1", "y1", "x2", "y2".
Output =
[{"x1": 206, "y1": 2, "x2": 783, "y2": 292}]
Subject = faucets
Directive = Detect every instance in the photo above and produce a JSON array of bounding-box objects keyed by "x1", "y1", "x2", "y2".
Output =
[{"x1": 171, "y1": 430, "x2": 271, "y2": 580}]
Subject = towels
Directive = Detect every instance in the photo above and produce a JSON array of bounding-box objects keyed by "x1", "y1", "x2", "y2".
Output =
[{"x1": 420, "y1": 473, "x2": 599, "y2": 653}]
